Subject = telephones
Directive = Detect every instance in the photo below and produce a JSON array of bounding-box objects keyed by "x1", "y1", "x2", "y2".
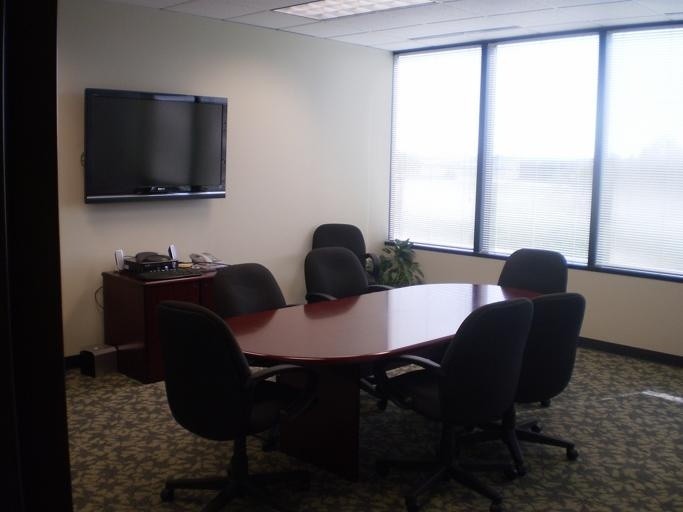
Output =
[{"x1": 190, "y1": 251, "x2": 223, "y2": 266}]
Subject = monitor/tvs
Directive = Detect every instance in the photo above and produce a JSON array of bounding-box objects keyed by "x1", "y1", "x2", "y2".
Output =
[{"x1": 83, "y1": 88, "x2": 228, "y2": 204}]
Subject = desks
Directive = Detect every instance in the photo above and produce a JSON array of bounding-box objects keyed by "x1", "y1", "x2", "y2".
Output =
[
  {"x1": 221, "y1": 282, "x2": 542, "y2": 482},
  {"x1": 101, "y1": 264, "x2": 215, "y2": 384}
]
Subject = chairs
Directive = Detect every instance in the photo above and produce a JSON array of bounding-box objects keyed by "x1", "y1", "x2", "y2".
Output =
[
  {"x1": 312, "y1": 223, "x2": 381, "y2": 286},
  {"x1": 156, "y1": 298, "x2": 315, "y2": 512},
  {"x1": 515, "y1": 292, "x2": 585, "y2": 404},
  {"x1": 305, "y1": 245, "x2": 393, "y2": 302},
  {"x1": 403, "y1": 297, "x2": 533, "y2": 510},
  {"x1": 498, "y1": 249, "x2": 568, "y2": 407},
  {"x1": 211, "y1": 263, "x2": 286, "y2": 319}
]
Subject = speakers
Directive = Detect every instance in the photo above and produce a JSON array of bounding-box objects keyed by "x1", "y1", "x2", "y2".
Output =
[
  {"x1": 168, "y1": 244, "x2": 179, "y2": 265},
  {"x1": 115, "y1": 249, "x2": 124, "y2": 272}
]
